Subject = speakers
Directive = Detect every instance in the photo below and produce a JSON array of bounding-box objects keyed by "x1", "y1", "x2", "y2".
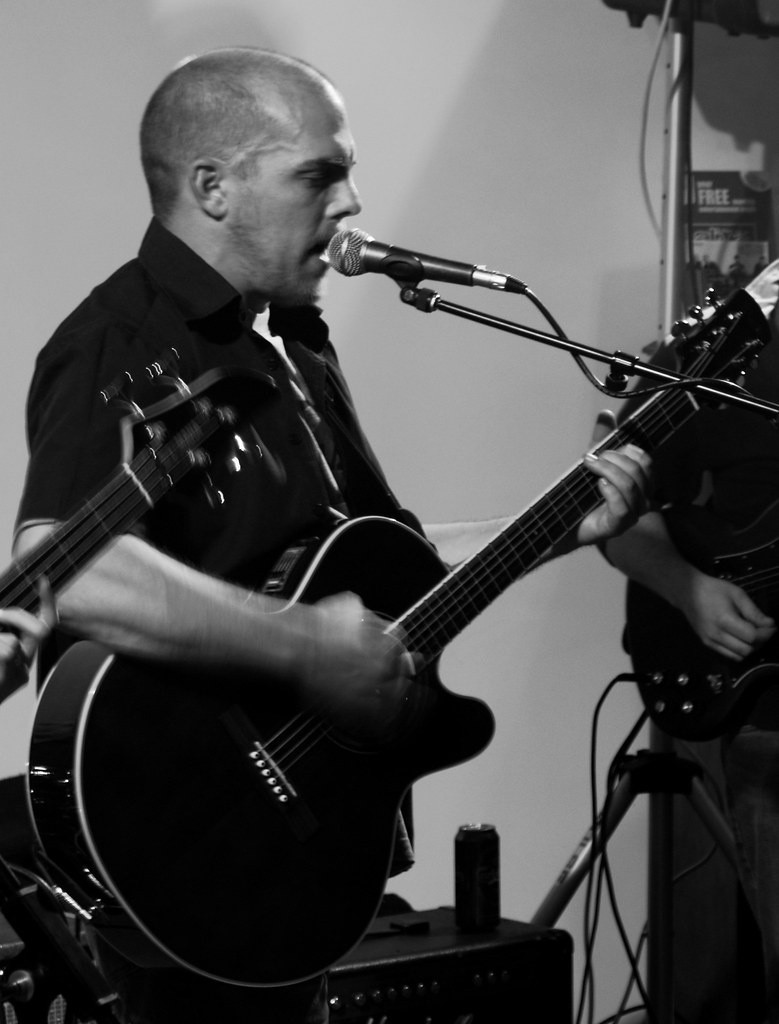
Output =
[{"x1": 313, "y1": 902, "x2": 574, "y2": 1024}]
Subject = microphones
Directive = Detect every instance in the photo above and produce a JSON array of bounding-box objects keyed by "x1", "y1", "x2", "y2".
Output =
[{"x1": 328, "y1": 227, "x2": 529, "y2": 295}]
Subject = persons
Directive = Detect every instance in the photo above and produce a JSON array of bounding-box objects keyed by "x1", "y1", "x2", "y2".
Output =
[
  {"x1": 13, "y1": 49, "x2": 653, "y2": 1024},
  {"x1": 597, "y1": 260, "x2": 779, "y2": 1024}
]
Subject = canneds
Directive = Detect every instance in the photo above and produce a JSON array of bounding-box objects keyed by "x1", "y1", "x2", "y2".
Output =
[{"x1": 454, "y1": 823, "x2": 501, "y2": 929}]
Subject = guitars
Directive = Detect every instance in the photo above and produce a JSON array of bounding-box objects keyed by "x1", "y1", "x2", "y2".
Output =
[
  {"x1": 623, "y1": 494, "x2": 779, "y2": 743},
  {"x1": 23, "y1": 278, "x2": 773, "y2": 994},
  {"x1": 1, "y1": 345, "x2": 281, "y2": 704}
]
